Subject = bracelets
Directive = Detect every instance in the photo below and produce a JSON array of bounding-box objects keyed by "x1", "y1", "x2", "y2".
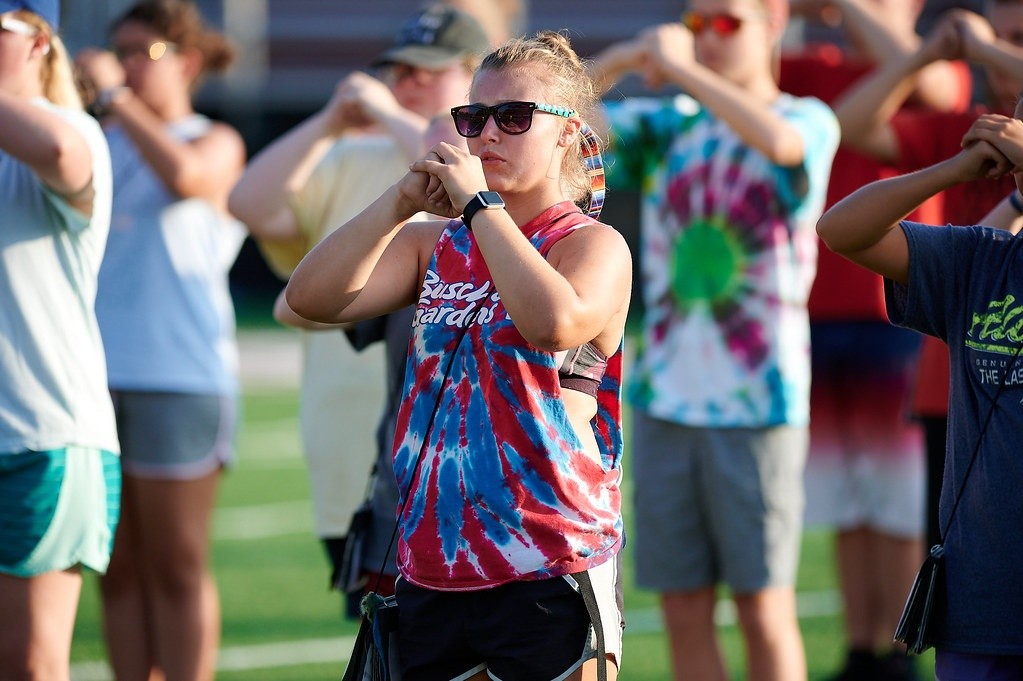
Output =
[{"x1": 98, "y1": 84, "x2": 133, "y2": 109}]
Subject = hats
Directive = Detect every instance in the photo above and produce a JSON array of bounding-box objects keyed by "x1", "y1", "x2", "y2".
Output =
[{"x1": 371, "y1": 11, "x2": 493, "y2": 72}]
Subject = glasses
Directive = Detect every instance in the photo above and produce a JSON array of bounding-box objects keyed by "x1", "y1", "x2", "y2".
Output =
[
  {"x1": 114, "y1": 42, "x2": 174, "y2": 58},
  {"x1": 682, "y1": 10, "x2": 766, "y2": 34},
  {"x1": 451, "y1": 101, "x2": 571, "y2": 139}
]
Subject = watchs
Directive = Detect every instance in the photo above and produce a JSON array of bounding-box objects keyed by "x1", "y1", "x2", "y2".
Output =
[{"x1": 460, "y1": 191, "x2": 507, "y2": 232}]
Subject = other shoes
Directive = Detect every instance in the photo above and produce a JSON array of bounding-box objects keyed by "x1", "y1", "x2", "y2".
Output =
[{"x1": 833, "y1": 643, "x2": 914, "y2": 681}]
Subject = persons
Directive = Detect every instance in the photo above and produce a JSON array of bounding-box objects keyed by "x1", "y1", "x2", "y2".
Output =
[
  {"x1": 0, "y1": 0, "x2": 122, "y2": 681},
  {"x1": 580, "y1": 0, "x2": 841, "y2": 681},
  {"x1": 816, "y1": 96, "x2": 1023, "y2": 681},
  {"x1": 227, "y1": 5, "x2": 494, "y2": 621},
  {"x1": 73, "y1": 0, "x2": 250, "y2": 681},
  {"x1": 284, "y1": 30, "x2": 632, "y2": 681},
  {"x1": 778, "y1": 0, "x2": 1023, "y2": 681}
]
